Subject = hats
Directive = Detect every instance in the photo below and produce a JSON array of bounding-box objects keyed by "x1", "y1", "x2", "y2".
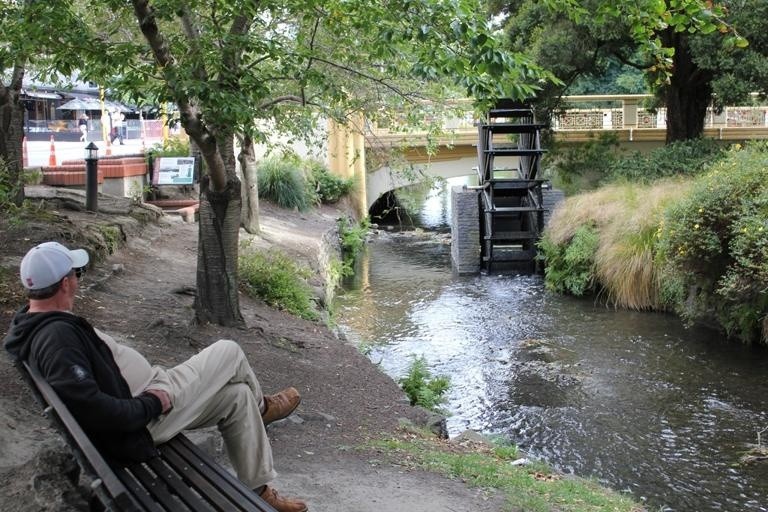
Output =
[{"x1": 19, "y1": 241, "x2": 91, "y2": 291}]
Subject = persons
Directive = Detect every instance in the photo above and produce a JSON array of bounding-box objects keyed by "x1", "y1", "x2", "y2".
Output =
[
  {"x1": 110, "y1": 106, "x2": 125, "y2": 146},
  {"x1": 101, "y1": 108, "x2": 111, "y2": 144},
  {"x1": 77, "y1": 113, "x2": 90, "y2": 142},
  {"x1": 0, "y1": 237, "x2": 310, "y2": 510}
]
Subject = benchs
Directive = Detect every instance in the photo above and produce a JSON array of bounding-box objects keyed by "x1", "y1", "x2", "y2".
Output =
[{"x1": 22, "y1": 359, "x2": 280, "y2": 511}]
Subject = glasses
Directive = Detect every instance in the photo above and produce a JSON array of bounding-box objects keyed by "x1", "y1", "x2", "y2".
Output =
[{"x1": 69, "y1": 268, "x2": 82, "y2": 278}]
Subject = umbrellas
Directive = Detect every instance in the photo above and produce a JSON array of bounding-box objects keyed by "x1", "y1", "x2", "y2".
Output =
[{"x1": 56, "y1": 96, "x2": 134, "y2": 130}]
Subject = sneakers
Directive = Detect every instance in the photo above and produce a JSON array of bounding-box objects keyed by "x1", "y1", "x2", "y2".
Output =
[
  {"x1": 258, "y1": 483, "x2": 308, "y2": 512},
  {"x1": 262, "y1": 386, "x2": 301, "y2": 427}
]
